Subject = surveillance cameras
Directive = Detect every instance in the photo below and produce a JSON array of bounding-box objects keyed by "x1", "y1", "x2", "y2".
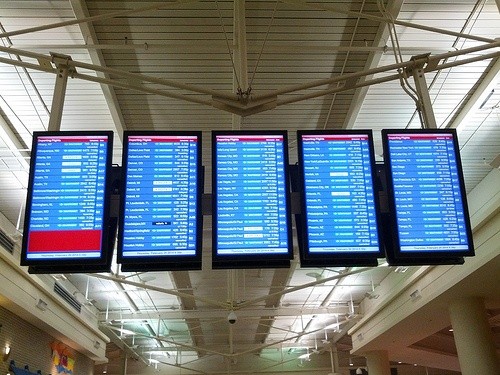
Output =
[{"x1": 228, "y1": 311, "x2": 237, "y2": 324}]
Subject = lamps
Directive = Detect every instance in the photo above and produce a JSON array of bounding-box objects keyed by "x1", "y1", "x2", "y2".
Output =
[
  {"x1": 9, "y1": 187, "x2": 27, "y2": 241},
  {"x1": 5, "y1": 343, "x2": 10, "y2": 355},
  {"x1": 49, "y1": 274, "x2": 96, "y2": 307},
  {"x1": 102, "y1": 280, "x2": 160, "y2": 369},
  {"x1": 334, "y1": 265, "x2": 408, "y2": 334},
  {"x1": 297, "y1": 328, "x2": 330, "y2": 368}
]
